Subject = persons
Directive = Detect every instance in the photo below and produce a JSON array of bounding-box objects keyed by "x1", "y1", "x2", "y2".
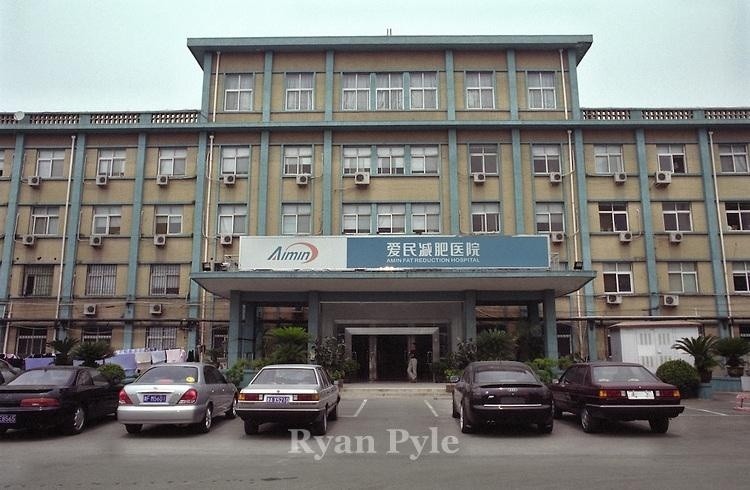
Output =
[
  {"x1": 572, "y1": 351, "x2": 584, "y2": 363},
  {"x1": 308, "y1": 346, "x2": 317, "y2": 365},
  {"x1": 407, "y1": 343, "x2": 417, "y2": 383},
  {"x1": 180, "y1": 344, "x2": 200, "y2": 363}
]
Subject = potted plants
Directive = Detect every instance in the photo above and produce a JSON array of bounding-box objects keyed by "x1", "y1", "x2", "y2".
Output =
[{"x1": 670, "y1": 334, "x2": 749, "y2": 383}]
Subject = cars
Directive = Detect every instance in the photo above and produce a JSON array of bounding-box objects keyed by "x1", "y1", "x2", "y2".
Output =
[
  {"x1": 235, "y1": 364, "x2": 341, "y2": 437},
  {"x1": 117, "y1": 362, "x2": 238, "y2": 433},
  {"x1": 451, "y1": 361, "x2": 555, "y2": 434},
  {"x1": 551, "y1": 362, "x2": 685, "y2": 434},
  {"x1": 0, "y1": 358, "x2": 124, "y2": 437}
]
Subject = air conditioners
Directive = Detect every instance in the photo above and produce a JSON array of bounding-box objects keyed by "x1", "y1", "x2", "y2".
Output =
[
  {"x1": 551, "y1": 232, "x2": 564, "y2": 242},
  {"x1": 149, "y1": 301, "x2": 162, "y2": 314},
  {"x1": 24, "y1": 234, "x2": 103, "y2": 247},
  {"x1": 28, "y1": 176, "x2": 41, "y2": 185},
  {"x1": 619, "y1": 231, "x2": 683, "y2": 242},
  {"x1": 154, "y1": 234, "x2": 232, "y2": 244},
  {"x1": 95, "y1": 175, "x2": 169, "y2": 186},
  {"x1": 474, "y1": 173, "x2": 486, "y2": 180},
  {"x1": 614, "y1": 171, "x2": 672, "y2": 185},
  {"x1": 83, "y1": 302, "x2": 98, "y2": 315},
  {"x1": 354, "y1": 172, "x2": 370, "y2": 185},
  {"x1": 296, "y1": 174, "x2": 308, "y2": 185},
  {"x1": 224, "y1": 174, "x2": 236, "y2": 184},
  {"x1": 606, "y1": 294, "x2": 678, "y2": 306},
  {"x1": 550, "y1": 172, "x2": 563, "y2": 183}
]
